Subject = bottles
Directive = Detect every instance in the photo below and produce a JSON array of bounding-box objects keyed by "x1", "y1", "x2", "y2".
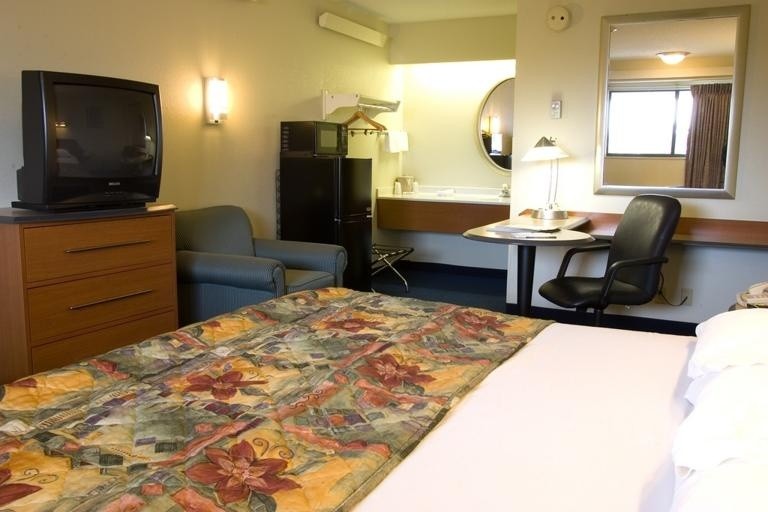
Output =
[
  {"x1": 394, "y1": 179, "x2": 401, "y2": 196},
  {"x1": 413, "y1": 179, "x2": 419, "y2": 192}
]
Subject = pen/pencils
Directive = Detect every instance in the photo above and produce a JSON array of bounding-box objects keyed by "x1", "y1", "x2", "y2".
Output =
[{"x1": 526, "y1": 237, "x2": 556, "y2": 238}]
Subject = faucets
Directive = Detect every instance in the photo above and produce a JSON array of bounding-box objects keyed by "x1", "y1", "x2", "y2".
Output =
[{"x1": 499, "y1": 183, "x2": 510, "y2": 197}]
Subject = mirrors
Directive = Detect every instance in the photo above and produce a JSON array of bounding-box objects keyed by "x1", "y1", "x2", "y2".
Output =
[
  {"x1": 594, "y1": 5, "x2": 751, "y2": 200},
  {"x1": 477, "y1": 78, "x2": 514, "y2": 170}
]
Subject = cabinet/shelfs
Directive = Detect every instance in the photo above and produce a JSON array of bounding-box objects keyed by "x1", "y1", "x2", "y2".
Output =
[{"x1": 0, "y1": 207, "x2": 181, "y2": 383}]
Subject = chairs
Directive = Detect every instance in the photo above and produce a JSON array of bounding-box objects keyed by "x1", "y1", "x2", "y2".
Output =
[
  {"x1": 539, "y1": 194, "x2": 679, "y2": 325},
  {"x1": 175, "y1": 204, "x2": 349, "y2": 319}
]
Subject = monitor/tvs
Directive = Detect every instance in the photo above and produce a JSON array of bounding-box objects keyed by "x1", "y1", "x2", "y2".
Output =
[{"x1": 16, "y1": 70, "x2": 163, "y2": 210}]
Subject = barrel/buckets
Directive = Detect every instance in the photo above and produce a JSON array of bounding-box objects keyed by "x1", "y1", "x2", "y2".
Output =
[{"x1": 397, "y1": 175, "x2": 415, "y2": 193}]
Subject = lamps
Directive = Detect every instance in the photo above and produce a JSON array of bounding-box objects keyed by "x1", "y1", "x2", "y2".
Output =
[
  {"x1": 520, "y1": 137, "x2": 567, "y2": 219},
  {"x1": 654, "y1": 51, "x2": 690, "y2": 66}
]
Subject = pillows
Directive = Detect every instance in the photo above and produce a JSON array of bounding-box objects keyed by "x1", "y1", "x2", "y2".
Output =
[{"x1": 677, "y1": 307, "x2": 768, "y2": 512}]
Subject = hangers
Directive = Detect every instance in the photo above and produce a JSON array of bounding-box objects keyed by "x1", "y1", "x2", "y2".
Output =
[{"x1": 344, "y1": 105, "x2": 387, "y2": 135}]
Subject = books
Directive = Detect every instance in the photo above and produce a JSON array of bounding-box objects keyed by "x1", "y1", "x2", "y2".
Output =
[
  {"x1": 485, "y1": 216, "x2": 562, "y2": 234},
  {"x1": 509, "y1": 232, "x2": 557, "y2": 241}
]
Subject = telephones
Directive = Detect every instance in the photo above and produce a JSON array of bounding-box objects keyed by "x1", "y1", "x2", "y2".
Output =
[{"x1": 740, "y1": 282, "x2": 768, "y2": 306}]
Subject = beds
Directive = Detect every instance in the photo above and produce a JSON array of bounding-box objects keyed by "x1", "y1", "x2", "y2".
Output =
[{"x1": 0, "y1": 285, "x2": 768, "y2": 512}]
